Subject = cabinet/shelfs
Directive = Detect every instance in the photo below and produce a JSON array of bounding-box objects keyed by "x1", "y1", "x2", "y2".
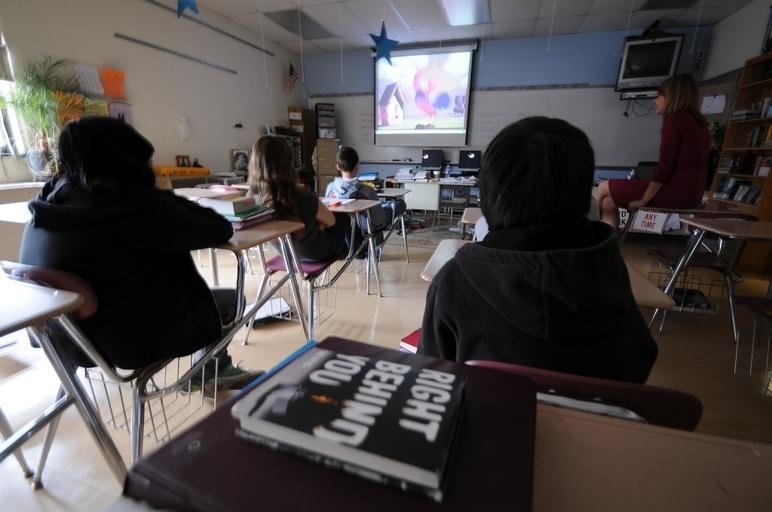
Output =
[
  {"x1": 708, "y1": 53, "x2": 771, "y2": 280},
  {"x1": 382, "y1": 178, "x2": 480, "y2": 222},
  {"x1": 312, "y1": 138, "x2": 344, "y2": 197},
  {"x1": 315, "y1": 103, "x2": 337, "y2": 139}
]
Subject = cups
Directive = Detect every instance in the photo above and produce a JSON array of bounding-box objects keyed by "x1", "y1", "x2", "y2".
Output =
[{"x1": 433, "y1": 171, "x2": 441, "y2": 181}]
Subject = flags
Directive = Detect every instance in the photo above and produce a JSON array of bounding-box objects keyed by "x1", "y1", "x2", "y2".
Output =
[{"x1": 288, "y1": 64, "x2": 300, "y2": 94}]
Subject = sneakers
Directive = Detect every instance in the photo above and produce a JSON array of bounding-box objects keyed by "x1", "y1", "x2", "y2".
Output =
[
  {"x1": 205, "y1": 360, "x2": 266, "y2": 398},
  {"x1": 182, "y1": 379, "x2": 201, "y2": 391}
]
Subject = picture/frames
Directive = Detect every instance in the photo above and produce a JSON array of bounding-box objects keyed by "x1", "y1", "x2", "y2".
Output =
[
  {"x1": 231, "y1": 148, "x2": 250, "y2": 171},
  {"x1": 176, "y1": 155, "x2": 190, "y2": 168}
]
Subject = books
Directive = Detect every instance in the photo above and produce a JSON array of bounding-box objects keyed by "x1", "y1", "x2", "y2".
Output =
[
  {"x1": 173, "y1": 187, "x2": 276, "y2": 230},
  {"x1": 394, "y1": 167, "x2": 432, "y2": 182},
  {"x1": 125, "y1": 335, "x2": 536, "y2": 512},
  {"x1": 712, "y1": 96, "x2": 772, "y2": 208}
]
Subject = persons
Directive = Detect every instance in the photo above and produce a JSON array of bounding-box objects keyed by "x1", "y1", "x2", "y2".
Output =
[
  {"x1": 20, "y1": 116, "x2": 267, "y2": 395},
  {"x1": 247, "y1": 135, "x2": 366, "y2": 263},
  {"x1": 415, "y1": 116, "x2": 659, "y2": 386},
  {"x1": 235, "y1": 152, "x2": 249, "y2": 172},
  {"x1": 325, "y1": 146, "x2": 386, "y2": 263},
  {"x1": 596, "y1": 75, "x2": 710, "y2": 245}
]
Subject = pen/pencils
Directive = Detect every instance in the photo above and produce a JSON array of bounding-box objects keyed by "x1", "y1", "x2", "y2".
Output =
[{"x1": 328, "y1": 201, "x2": 342, "y2": 208}]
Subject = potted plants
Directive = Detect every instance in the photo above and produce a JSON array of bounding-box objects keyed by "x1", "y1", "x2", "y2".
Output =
[{"x1": 0, "y1": 56, "x2": 107, "y2": 182}]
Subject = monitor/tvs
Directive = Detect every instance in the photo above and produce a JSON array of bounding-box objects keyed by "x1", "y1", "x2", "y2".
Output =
[
  {"x1": 616, "y1": 33, "x2": 685, "y2": 100},
  {"x1": 459, "y1": 151, "x2": 481, "y2": 171},
  {"x1": 422, "y1": 150, "x2": 444, "y2": 170}
]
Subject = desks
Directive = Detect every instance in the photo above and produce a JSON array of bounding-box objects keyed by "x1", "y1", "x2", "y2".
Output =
[
  {"x1": 419, "y1": 239, "x2": 675, "y2": 309},
  {"x1": 121, "y1": 340, "x2": 771, "y2": 512},
  {"x1": 212, "y1": 171, "x2": 244, "y2": 185},
  {"x1": 153, "y1": 165, "x2": 210, "y2": 190},
  {"x1": 596, "y1": 168, "x2": 771, "y2": 375},
  {"x1": 459, "y1": 208, "x2": 484, "y2": 239}
]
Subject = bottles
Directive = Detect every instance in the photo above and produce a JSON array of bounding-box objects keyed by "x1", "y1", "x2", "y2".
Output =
[{"x1": 445, "y1": 165, "x2": 451, "y2": 178}]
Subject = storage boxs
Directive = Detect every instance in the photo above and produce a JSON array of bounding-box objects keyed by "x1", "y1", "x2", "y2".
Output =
[{"x1": 287, "y1": 106, "x2": 317, "y2": 133}]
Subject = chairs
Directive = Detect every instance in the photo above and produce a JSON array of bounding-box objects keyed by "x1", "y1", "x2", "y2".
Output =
[
  {"x1": 463, "y1": 360, "x2": 703, "y2": 433},
  {"x1": 404, "y1": 183, "x2": 442, "y2": 232}
]
